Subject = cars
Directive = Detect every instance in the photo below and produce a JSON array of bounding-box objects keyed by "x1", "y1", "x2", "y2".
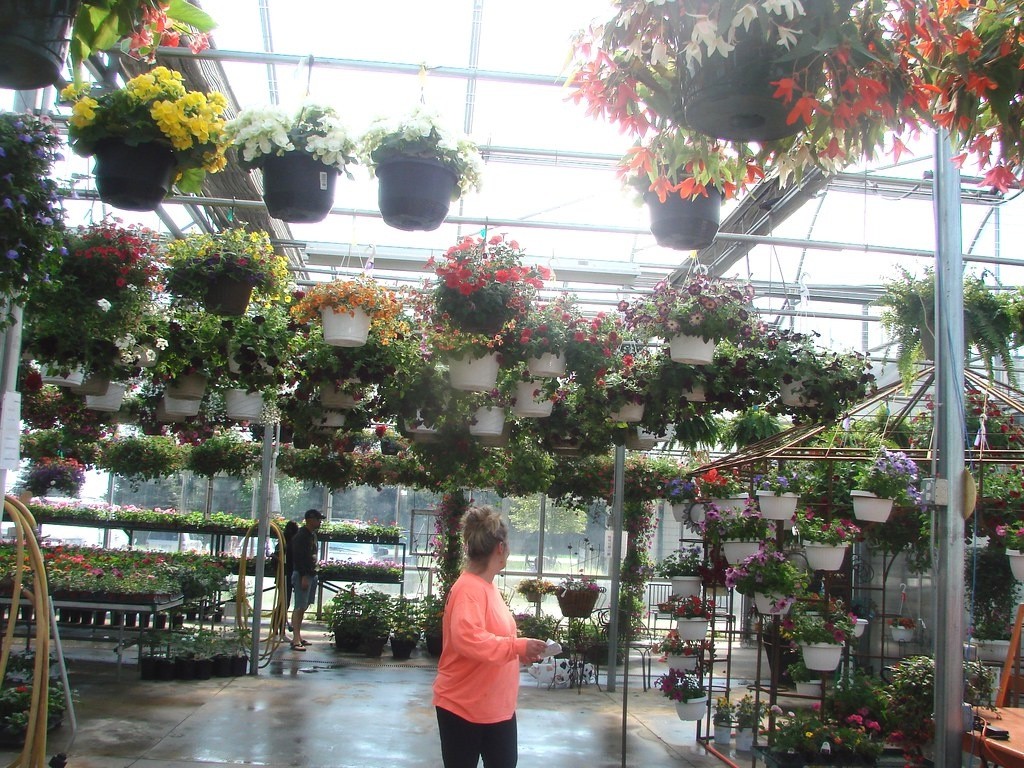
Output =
[
  {"x1": 236, "y1": 535, "x2": 275, "y2": 557},
  {"x1": 318, "y1": 518, "x2": 389, "y2": 565}
]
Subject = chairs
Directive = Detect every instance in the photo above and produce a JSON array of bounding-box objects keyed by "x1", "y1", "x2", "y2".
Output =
[{"x1": 578, "y1": 607, "x2": 652, "y2": 693}]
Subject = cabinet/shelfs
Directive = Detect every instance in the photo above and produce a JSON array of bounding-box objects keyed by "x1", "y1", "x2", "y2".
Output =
[
  {"x1": 751, "y1": 547, "x2": 853, "y2": 768},
  {"x1": 0, "y1": 519, "x2": 215, "y2": 617},
  {"x1": 0, "y1": 598, "x2": 203, "y2": 679},
  {"x1": 682, "y1": 537, "x2": 734, "y2": 742},
  {"x1": 219, "y1": 534, "x2": 406, "y2": 622}
]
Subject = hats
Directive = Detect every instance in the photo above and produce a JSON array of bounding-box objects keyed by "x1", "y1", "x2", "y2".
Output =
[{"x1": 305, "y1": 509, "x2": 326, "y2": 519}]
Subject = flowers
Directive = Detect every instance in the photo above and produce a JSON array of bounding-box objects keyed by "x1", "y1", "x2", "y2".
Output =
[{"x1": 0, "y1": 1, "x2": 1024, "y2": 768}]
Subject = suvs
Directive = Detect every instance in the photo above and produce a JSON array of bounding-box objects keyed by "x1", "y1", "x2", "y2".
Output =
[{"x1": 146, "y1": 530, "x2": 204, "y2": 556}]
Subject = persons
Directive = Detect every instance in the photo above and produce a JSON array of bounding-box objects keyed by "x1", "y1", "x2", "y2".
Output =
[
  {"x1": 270, "y1": 520, "x2": 292, "y2": 642},
  {"x1": 290, "y1": 509, "x2": 327, "y2": 651},
  {"x1": 432, "y1": 504, "x2": 548, "y2": 768}
]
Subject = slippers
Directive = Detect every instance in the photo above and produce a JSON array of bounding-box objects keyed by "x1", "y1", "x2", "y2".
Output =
[
  {"x1": 291, "y1": 641, "x2": 306, "y2": 651},
  {"x1": 301, "y1": 640, "x2": 312, "y2": 645}
]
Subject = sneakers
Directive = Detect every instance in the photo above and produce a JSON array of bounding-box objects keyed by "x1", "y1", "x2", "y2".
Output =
[
  {"x1": 283, "y1": 635, "x2": 293, "y2": 642},
  {"x1": 273, "y1": 635, "x2": 284, "y2": 642}
]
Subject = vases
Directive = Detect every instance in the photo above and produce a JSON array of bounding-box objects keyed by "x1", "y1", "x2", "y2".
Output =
[
  {"x1": 526, "y1": 352, "x2": 566, "y2": 377},
  {"x1": 261, "y1": 155, "x2": 336, "y2": 223},
  {"x1": 200, "y1": 273, "x2": 252, "y2": 316},
  {"x1": 470, "y1": 405, "x2": 503, "y2": 438},
  {"x1": 450, "y1": 350, "x2": 499, "y2": 392},
  {"x1": 644, "y1": 182, "x2": 722, "y2": 250},
  {"x1": 515, "y1": 377, "x2": 553, "y2": 417},
  {"x1": 670, "y1": 330, "x2": 716, "y2": 366},
  {"x1": 377, "y1": 160, "x2": 458, "y2": 232},
  {"x1": 94, "y1": 135, "x2": 174, "y2": 209},
  {"x1": 1, "y1": 1, "x2": 74, "y2": 90},
  {"x1": 319, "y1": 303, "x2": 367, "y2": 345},
  {"x1": 674, "y1": 18, "x2": 816, "y2": 139}
]
